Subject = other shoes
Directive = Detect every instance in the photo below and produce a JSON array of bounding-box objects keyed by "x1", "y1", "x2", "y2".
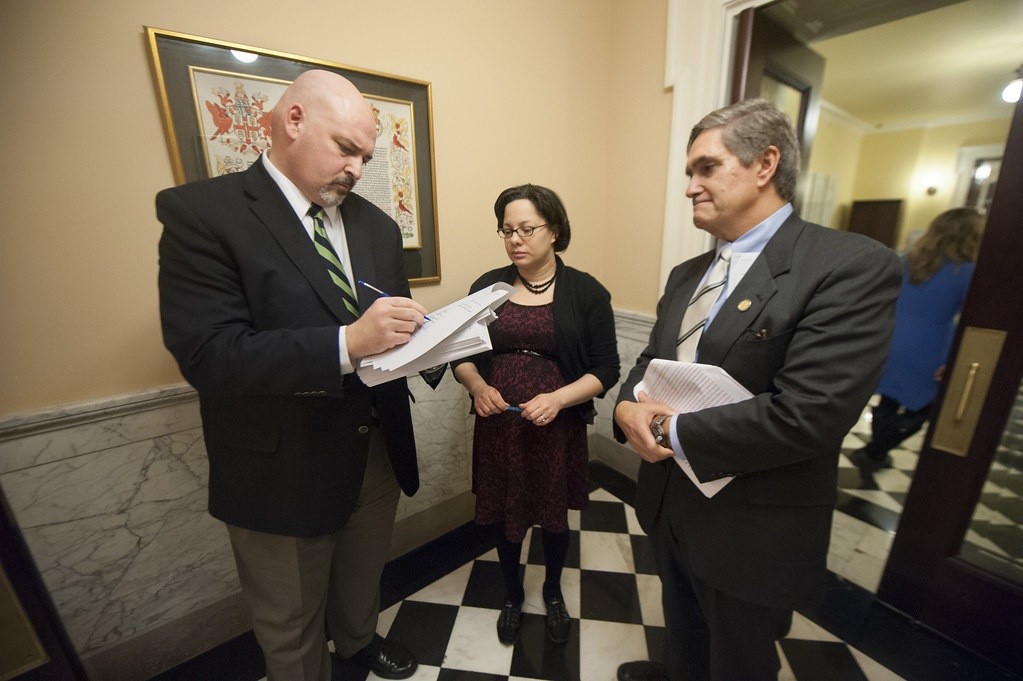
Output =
[
  {"x1": 617, "y1": 659, "x2": 667, "y2": 681},
  {"x1": 851, "y1": 448, "x2": 892, "y2": 467}
]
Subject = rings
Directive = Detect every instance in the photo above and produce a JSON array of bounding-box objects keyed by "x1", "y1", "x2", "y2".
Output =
[{"x1": 540, "y1": 416, "x2": 545, "y2": 422}]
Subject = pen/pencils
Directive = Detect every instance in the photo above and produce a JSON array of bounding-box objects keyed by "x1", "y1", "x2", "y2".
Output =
[
  {"x1": 505, "y1": 406, "x2": 522, "y2": 412},
  {"x1": 358, "y1": 280, "x2": 434, "y2": 323}
]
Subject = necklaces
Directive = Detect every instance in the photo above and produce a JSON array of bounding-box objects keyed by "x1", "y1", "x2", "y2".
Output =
[{"x1": 516, "y1": 270, "x2": 556, "y2": 294}]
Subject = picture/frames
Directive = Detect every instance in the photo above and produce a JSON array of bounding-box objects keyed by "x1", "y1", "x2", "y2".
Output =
[
  {"x1": 964, "y1": 154, "x2": 1003, "y2": 215},
  {"x1": 141, "y1": 23, "x2": 441, "y2": 289}
]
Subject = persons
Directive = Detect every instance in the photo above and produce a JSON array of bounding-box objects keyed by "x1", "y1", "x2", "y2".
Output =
[
  {"x1": 613, "y1": 97, "x2": 901, "y2": 680},
  {"x1": 155, "y1": 68, "x2": 447, "y2": 681},
  {"x1": 449, "y1": 183, "x2": 621, "y2": 646},
  {"x1": 852, "y1": 207, "x2": 981, "y2": 470}
]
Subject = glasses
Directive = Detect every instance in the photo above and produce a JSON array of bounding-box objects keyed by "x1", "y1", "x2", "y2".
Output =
[
  {"x1": 497, "y1": 224, "x2": 548, "y2": 238},
  {"x1": 743, "y1": 328, "x2": 767, "y2": 341}
]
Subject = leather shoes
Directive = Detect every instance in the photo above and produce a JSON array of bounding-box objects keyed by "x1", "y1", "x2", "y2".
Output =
[{"x1": 335, "y1": 634, "x2": 418, "y2": 680}]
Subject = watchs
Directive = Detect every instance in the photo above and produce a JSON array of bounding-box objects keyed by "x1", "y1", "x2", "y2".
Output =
[{"x1": 647, "y1": 414, "x2": 670, "y2": 450}]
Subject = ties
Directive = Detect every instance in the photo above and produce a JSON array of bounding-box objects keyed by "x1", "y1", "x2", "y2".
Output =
[
  {"x1": 307, "y1": 202, "x2": 361, "y2": 322},
  {"x1": 676, "y1": 249, "x2": 732, "y2": 363}
]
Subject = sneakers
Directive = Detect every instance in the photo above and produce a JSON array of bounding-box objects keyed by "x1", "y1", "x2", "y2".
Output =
[
  {"x1": 496, "y1": 589, "x2": 525, "y2": 644},
  {"x1": 542, "y1": 581, "x2": 571, "y2": 643}
]
